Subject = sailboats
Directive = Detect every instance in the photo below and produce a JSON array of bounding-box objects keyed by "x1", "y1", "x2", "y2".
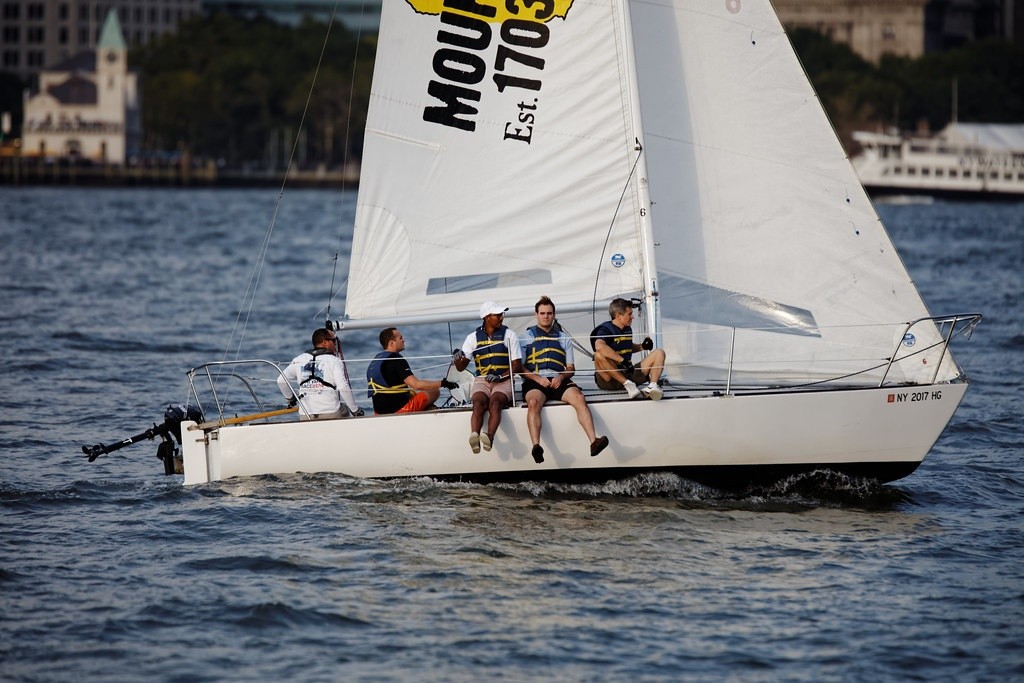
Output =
[{"x1": 181, "y1": 0, "x2": 984, "y2": 489}]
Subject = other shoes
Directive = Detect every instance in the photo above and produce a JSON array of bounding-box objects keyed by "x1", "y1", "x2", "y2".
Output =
[
  {"x1": 480, "y1": 432, "x2": 493, "y2": 452},
  {"x1": 469, "y1": 431, "x2": 481, "y2": 454},
  {"x1": 532, "y1": 444, "x2": 545, "y2": 463},
  {"x1": 590, "y1": 436, "x2": 609, "y2": 456}
]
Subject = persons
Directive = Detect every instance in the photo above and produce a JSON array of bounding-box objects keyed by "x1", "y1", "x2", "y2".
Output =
[
  {"x1": 453, "y1": 301, "x2": 522, "y2": 453},
  {"x1": 277, "y1": 329, "x2": 364, "y2": 421},
  {"x1": 590, "y1": 299, "x2": 666, "y2": 400},
  {"x1": 366, "y1": 327, "x2": 459, "y2": 415},
  {"x1": 518, "y1": 298, "x2": 609, "y2": 463}
]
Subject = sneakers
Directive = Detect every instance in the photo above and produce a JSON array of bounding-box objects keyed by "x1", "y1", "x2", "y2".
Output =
[
  {"x1": 640, "y1": 383, "x2": 663, "y2": 401},
  {"x1": 624, "y1": 381, "x2": 641, "y2": 400}
]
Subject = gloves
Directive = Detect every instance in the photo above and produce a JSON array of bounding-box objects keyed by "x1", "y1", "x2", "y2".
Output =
[
  {"x1": 441, "y1": 378, "x2": 459, "y2": 390},
  {"x1": 351, "y1": 409, "x2": 364, "y2": 416},
  {"x1": 287, "y1": 394, "x2": 297, "y2": 406},
  {"x1": 642, "y1": 336, "x2": 653, "y2": 351},
  {"x1": 621, "y1": 357, "x2": 634, "y2": 374}
]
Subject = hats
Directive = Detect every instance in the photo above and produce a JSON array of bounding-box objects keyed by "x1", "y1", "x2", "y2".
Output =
[{"x1": 480, "y1": 301, "x2": 509, "y2": 319}]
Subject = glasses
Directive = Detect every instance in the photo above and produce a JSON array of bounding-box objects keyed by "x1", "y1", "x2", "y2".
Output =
[
  {"x1": 321, "y1": 337, "x2": 337, "y2": 345},
  {"x1": 540, "y1": 312, "x2": 553, "y2": 316}
]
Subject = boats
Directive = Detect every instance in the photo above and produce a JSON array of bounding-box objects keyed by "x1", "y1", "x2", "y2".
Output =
[{"x1": 847, "y1": 123, "x2": 1024, "y2": 202}]
